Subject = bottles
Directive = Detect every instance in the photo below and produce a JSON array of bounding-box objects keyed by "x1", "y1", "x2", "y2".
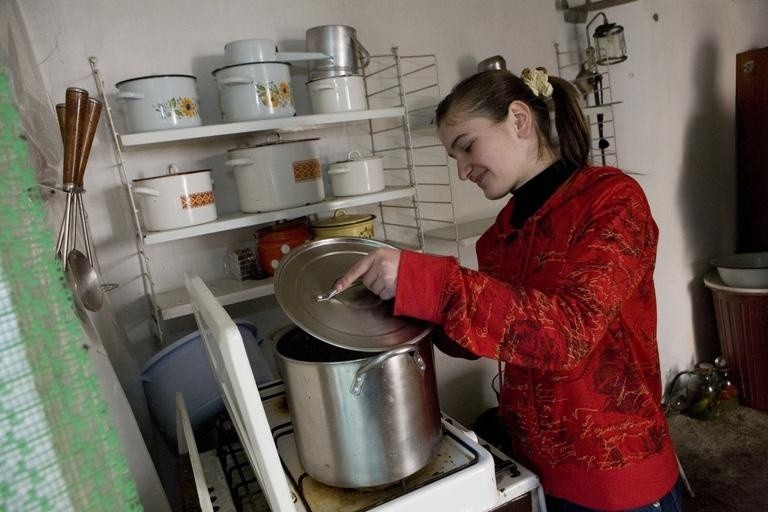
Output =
[{"x1": 707, "y1": 355, "x2": 740, "y2": 411}]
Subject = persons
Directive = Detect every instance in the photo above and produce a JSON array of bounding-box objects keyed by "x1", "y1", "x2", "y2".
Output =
[{"x1": 334, "y1": 71, "x2": 690, "y2": 511}]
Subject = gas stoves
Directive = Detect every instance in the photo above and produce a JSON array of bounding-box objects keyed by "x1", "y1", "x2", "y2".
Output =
[{"x1": 254, "y1": 372, "x2": 543, "y2": 511}]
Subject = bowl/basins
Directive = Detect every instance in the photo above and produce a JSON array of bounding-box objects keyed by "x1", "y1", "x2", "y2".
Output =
[
  {"x1": 708, "y1": 248, "x2": 768, "y2": 289},
  {"x1": 139, "y1": 317, "x2": 277, "y2": 449}
]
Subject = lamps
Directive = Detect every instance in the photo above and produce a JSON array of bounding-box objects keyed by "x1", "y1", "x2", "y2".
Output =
[{"x1": 584, "y1": 11, "x2": 630, "y2": 169}]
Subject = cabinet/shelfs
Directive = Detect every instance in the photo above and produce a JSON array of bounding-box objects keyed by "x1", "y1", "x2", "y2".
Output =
[{"x1": 120, "y1": 106, "x2": 423, "y2": 344}]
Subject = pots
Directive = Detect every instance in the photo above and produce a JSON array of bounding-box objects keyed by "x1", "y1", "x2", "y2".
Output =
[
  {"x1": 131, "y1": 163, "x2": 220, "y2": 233},
  {"x1": 257, "y1": 217, "x2": 313, "y2": 276},
  {"x1": 310, "y1": 209, "x2": 378, "y2": 242},
  {"x1": 221, "y1": 37, "x2": 334, "y2": 68},
  {"x1": 113, "y1": 73, "x2": 204, "y2": 135},
  {"x1": 223, "y1": 130, "x2": 326, "y2": 214},
  {"x1": 325, "y1": 150, "x2": 386, "y2": 198},
  {"x1": 304, "y1": 74, "x2": 370, "y2": 115},
  {"x1": 210, "y1": 60, "x2": 299, "y2": 125},
  {"x1": 269, "y1": 320, "x2": 447, "y2": 491}
]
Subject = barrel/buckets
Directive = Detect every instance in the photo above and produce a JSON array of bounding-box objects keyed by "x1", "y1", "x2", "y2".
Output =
[{"x1": 715, "y1": 356, "x2": 739, "y2": 411}]
